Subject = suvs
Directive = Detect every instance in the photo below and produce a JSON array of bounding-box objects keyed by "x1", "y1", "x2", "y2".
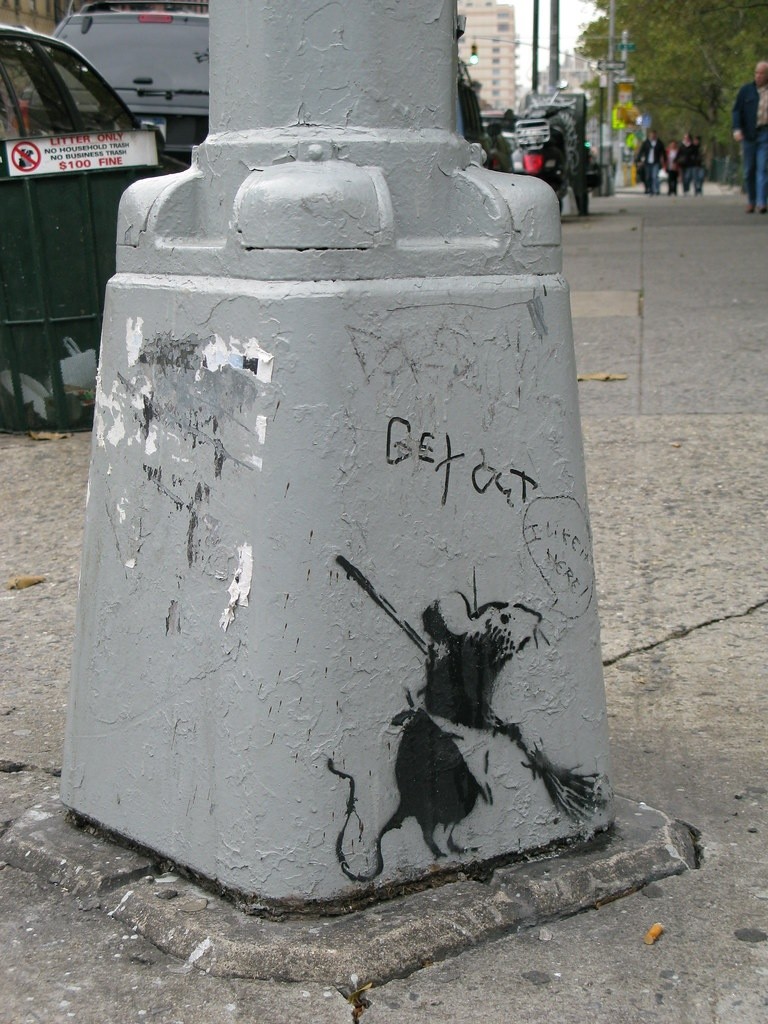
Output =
[{"x1": 24, "y1": 0, "x2": 210, "y2": 166}]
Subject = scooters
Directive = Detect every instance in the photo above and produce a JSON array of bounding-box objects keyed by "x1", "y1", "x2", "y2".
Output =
[{"x1": 503, "y1": 75, "x2": 590, "y2": 219}]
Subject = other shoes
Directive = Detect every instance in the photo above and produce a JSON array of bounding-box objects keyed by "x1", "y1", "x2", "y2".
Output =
[
  {"x1": 644, "y1": 190, "x2": 649, "y2": 194},
  {"x1": 745, "y1": 204, "x2": 753, "y2": 213},
  {"x1": 758, "y1": 205, "x2": 767, "y2": 213},
  {"x1": 668, "y1": 191, "x2": 672, "y2": 196}
]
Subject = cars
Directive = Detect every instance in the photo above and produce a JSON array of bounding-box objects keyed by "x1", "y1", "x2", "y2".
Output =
[{"x1": 0, "y1": 25, "x2": 194, "y2": 174}]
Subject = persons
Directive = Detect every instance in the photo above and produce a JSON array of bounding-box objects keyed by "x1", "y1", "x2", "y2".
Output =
[
  {"x1": 568, "y1": 142, "x2": 592, "y2": 218},
  {"x1": 481, "y1": 123, "x2": 515, "y2": 173},
  {"x1": 503, "y1": 109, "x2": 518, "y2": 132},
  {"x1": 730, "y1": 61, "x2": 768, "y2": 216},
  {"x1": 635, "y1": 129, "x2": 708, "y2": 198}
]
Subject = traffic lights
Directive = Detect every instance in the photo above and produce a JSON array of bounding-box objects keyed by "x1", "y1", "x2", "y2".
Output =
[{"x1": 470, "y1": 44, "x2": 478, "y2": 63}]
